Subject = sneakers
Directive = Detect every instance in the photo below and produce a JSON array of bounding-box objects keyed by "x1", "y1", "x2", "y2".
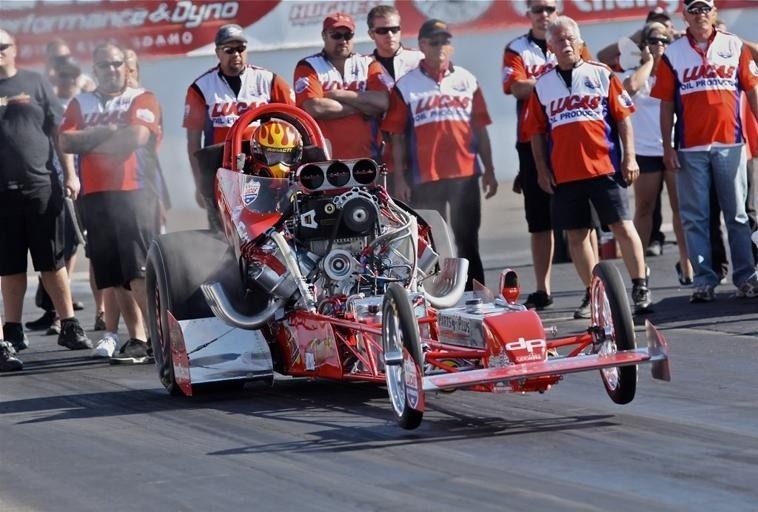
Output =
[
  {"x1": 73, "y1": 301, "x2": 82, "y2": 310},
  {"x1": 110, "y1": 339, "x2": 156, "y2": 365},
  {"x1": 646, "y1": 241, "x2": 663, "y2": 255},
  {"x1": 58, "y1": 319, "x2": 92, "y2": 350},
  {"x1": 522, "y1": 291, "x2": 553, "y2": 310},
  {"x1": 0, "y1": 341, "x2": 23, "y2": 371},
  {"x1": 95, "y1": 312, "x2": 107, "y2": 329},
  {"x1": 25, "y1": 311, "x2": 58, "y2": 330},
  {"x1": 736, "y1": 275, "x2": 757, "y2": 297},
  {"x1": 48, "y1": 318, "x2": 61, "y2": 334},
  {"x1": 716, "y1": 266, "x2": 728, "y2": 284},
  {"x1": 632, "y1": 286, "x2": 654, "y2": 313},
  {"x1": 690, "y1": 285, "x2": 716, "y2": 303},
  {"x1": 3, "y1": 323, "x2": 28, "y2": 350},
  {"x1": 92, "y1": 333, "x2": 119, "y2": 357},
  {"x1": 573, "y1": 293, "x2": 592, "y2": 319}
]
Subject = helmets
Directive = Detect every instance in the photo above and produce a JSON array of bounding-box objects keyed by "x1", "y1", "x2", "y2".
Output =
[{"x1": 252, "y1": 123, "x2": 302, "y2": 180}]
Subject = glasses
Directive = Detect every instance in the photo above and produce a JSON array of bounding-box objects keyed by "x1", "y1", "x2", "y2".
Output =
[
  {"x1": 687, "y1": 6, "x2": 712, "y2": 14},
  {"x1": 57, "y1": 71, "x2": 80, "y2": 79},
  {"x1": 370, "y1": 25, "x2": 401, "y2": 35},
  {"x1": 423, "y1": 37, "x2": 450, "y2": 45},
  {"x1": 526, "y1": 6, "x2": 557, "y2": 13},
  {"x1": 95, "y1": 61, "x2": 126, "y2": 68},
  {"x1": 325, "y1": 31, "x2": 354, "y2": 40},
  {"x1": 646, "y1": 37, "x2": 670, "y2": 43},
  {"x1": 53, "y1": 53, "x2": 74, "y2": 63},
  {"x1": 217, "y1": 44, "x2": 247, "y2": 54},
  {"x1": 0, "y1": 42, "x2": 14, "y2": 51}
]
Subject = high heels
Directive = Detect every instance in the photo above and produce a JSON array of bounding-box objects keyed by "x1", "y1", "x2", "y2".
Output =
[
  {"x1": 675, "y1": 262, "x2": 693, "y2": 285},
  {"x1": 645, "y1": 265, "x2": 651, "y2": 288}
]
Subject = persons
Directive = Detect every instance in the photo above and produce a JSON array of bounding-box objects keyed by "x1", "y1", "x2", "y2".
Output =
[
  {"x1": 503, "y1": 0, "x2": 599, "y2": 310},
  {"x1": 650, "y1": 0, "x2": 758, "y2": 301},
  {"x1": 380, "y1": 19, "x2": 496, "y2": 291},
  {"x1": 709, "y1": 20, "x2": 758, "y2": 285},
  {"x1": 246, "y1": 120, "x2": 323, "y2": 260},
  {"x1": 523, "y1": 16, "x2": 654, "y2": 319},
  {"x1": 620, "y1": 23, "x2": 694, "y2": 284},
  {"x1": 183, "y1": 24, "x2": 295, "y2": 209},
  {"x1": 26, "y1": 39, "x2": 95, "y2": 330},
  {"x1": 0, "y1": 28, "x2": 93, "y2": 350},
  {"x1": 58, "y1": 44, "x2": 160, "y2": 364},
  {"x1": 47, "y1": 55, "x2": 107, "y2": 335},
  {"x1": 92, "y1": 48, "x2": 172, "y2": 356},
  {"x1": 294, "y1": 13, "x2": 389, "y2": 190},
  {"x1": 597, "y1": 8, "x2": 686, "y2": 257},
  {"x1": 363, "y1": 5, "x2": 425, "y2": 83}
]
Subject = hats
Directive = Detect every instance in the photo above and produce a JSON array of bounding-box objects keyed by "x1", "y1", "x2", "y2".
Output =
[
  {"x1": 647, "y1": 7, "x2": 670, "y2": 19},
  {"x1": 419, "y1": 20, "x2": 451, "y2": 38},
  {"x1": 684, "y1": 0, "x2": 713, "y2": 9},
  {"x1": 323, "y1": 12, "x2": 355, "y2": 31},
  {"x1": 215, "y1": 25, "x2": 248, "y2": 43}
]
